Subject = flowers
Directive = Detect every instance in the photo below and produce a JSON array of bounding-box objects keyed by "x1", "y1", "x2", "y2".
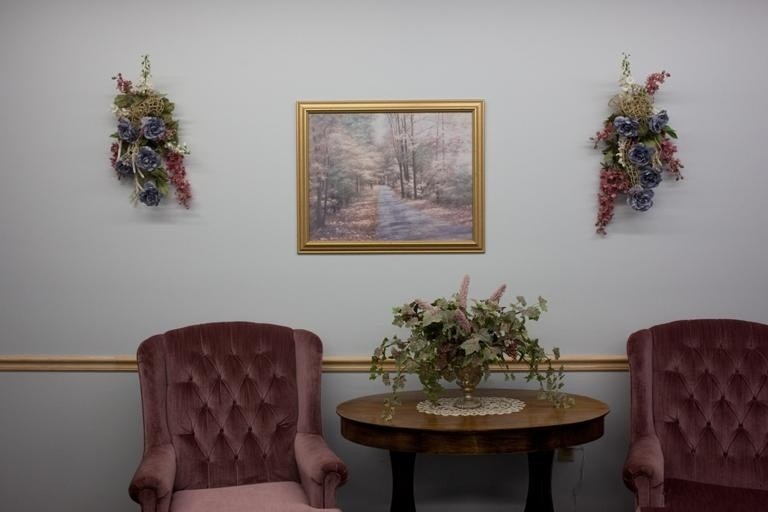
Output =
[
  {"x1": 590, "y1": 52, "x2": 684, "y2": 238},
  {"x1": 369, "y1": 274, "x2": 576, "y2": 419},
  {"x1": 111, "y1": 53, "x2": 195, "y2": 210}
]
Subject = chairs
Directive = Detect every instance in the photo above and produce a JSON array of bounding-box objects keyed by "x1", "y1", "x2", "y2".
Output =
[
  {"x1": 130, "y1": 321, "x2": 349, "y2": 512},
  {"x1": 626, "y1": 317, "x2": 765, "y2": 512}
]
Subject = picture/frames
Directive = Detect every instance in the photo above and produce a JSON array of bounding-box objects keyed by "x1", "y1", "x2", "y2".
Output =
[
  {"x1": 440, "y1": 344, "x2": 488, "y2": 411},
  {"x1": 293, "y1": 97, "x2": 488, "y2": 258}
]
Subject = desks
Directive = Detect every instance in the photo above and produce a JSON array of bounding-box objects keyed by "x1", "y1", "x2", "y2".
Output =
[{"x1": 337, "y1": 389, "x2": 612, "y2": 510}]
